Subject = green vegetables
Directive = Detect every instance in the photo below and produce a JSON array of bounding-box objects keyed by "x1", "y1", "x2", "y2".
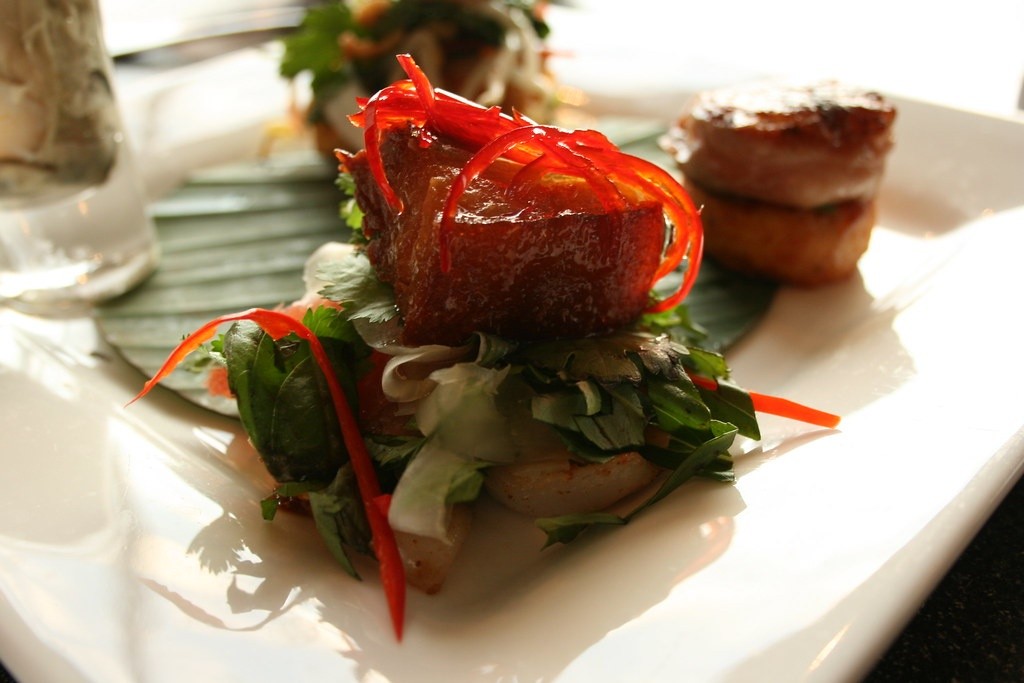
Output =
[
  {"x1": 280, "y1": 0, "x2": 577, "y2": 108},
  {"x1": 182, "y1": 166, "x2": 778, "y2": 582}
]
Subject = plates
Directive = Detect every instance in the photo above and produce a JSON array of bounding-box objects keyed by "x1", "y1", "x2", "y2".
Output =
[{"x1": 0, "y1": 0, "x2": 1024, "y2": 683}]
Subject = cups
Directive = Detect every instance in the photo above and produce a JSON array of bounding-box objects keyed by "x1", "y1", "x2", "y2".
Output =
[{"x1": 0, "y1": 0, "x2": 163, "y2": 305}]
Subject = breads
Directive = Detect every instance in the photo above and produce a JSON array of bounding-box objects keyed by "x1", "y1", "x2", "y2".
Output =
[{"x1": 659, "y1": 82, "x2": 898, "y2": 281}]
